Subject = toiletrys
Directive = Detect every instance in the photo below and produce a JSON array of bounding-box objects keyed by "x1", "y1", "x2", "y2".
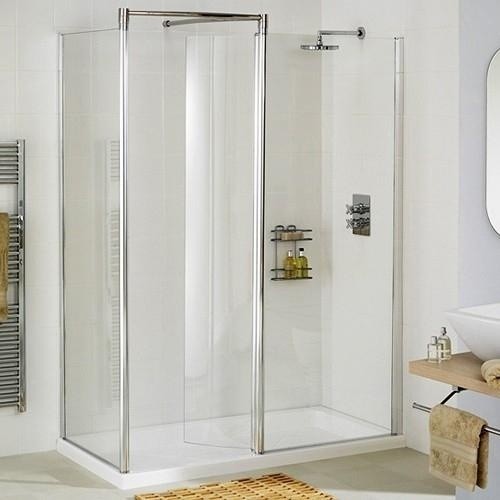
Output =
[
  {"x1": 284, "y1": 250, "x2": 296, "y2": 279},
  {"x1": 295, "y1": 248, "x2": 308, "y2": 277},
  {"x1": 438, "y1": 327, "x2": 451, "y2": 359}
]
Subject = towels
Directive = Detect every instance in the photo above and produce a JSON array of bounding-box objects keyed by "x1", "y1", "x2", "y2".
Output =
[
  {"x1": 0, "y1": 212, "x2": 10, "y2": 324},
  {"x1": 481, "y1": 359, "x2": 500, "y2": 391},
  {"x1": 428, "y1": 404, "x2": 488, "y2": 491}
]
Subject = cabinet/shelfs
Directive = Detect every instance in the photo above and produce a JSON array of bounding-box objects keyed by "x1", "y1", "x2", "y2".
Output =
[{"x1": 270, "y1": 225, "x2": 313, "y2": 280}]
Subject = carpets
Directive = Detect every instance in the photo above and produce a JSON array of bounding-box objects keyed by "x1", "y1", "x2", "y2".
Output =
[{"x1": 133, "y1": 471, "x2": 338, "y2": 500}]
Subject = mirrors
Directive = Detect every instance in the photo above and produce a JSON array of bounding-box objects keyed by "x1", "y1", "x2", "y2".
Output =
[{"x1": 485, "y1": 48, "x2": 500, "y2": 236}]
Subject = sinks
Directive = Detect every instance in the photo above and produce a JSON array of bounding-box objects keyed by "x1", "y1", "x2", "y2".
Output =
[{"x1": 446, "y1": 302, "x2": 500, "y2": 362}]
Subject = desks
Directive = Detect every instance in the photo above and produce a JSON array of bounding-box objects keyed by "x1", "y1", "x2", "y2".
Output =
[{"x1": 408, "y1": 351, "x2": 500, "y2": 400}]
